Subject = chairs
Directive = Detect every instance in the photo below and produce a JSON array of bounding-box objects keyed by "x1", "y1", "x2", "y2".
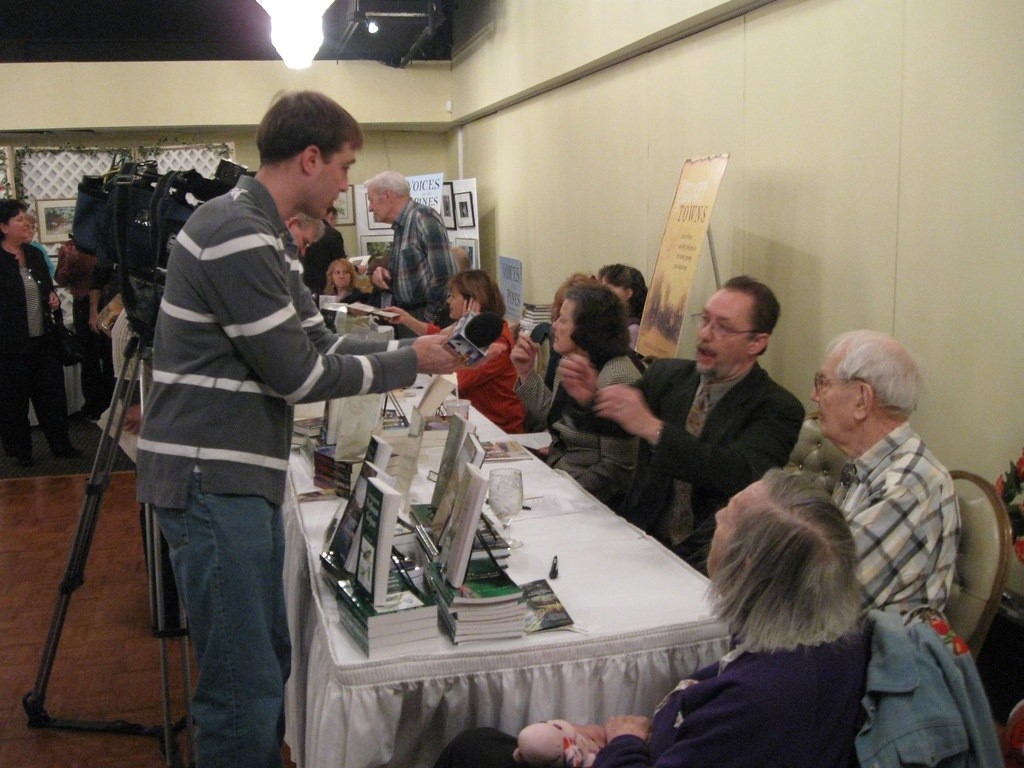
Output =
[
  {"x1": 782, "y1": 408, "x2": 853, "y2": 496},
  {"x1": 943, "y1": 469, "x2": 1008, "y2": 664},
  {"x1": 522, "y1": 321, "x2": 563, "y2": 433}
]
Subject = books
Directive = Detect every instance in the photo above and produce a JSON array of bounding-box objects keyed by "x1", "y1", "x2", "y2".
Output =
[
  {"x1": 291, "y1": 300, "x2": 532, "y2": 661},
  {"x1": 519, "y1": 302, "x2": 552, "y2": 330}
]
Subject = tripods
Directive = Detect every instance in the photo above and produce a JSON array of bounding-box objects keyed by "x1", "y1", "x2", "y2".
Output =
[{"x1": 22, "y1": 286, "x2": 196, "y2": 768}]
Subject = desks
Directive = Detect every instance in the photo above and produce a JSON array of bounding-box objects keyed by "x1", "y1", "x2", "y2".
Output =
[{"x1": 277, "y1": 336, "x2": 732, "y2": 768}]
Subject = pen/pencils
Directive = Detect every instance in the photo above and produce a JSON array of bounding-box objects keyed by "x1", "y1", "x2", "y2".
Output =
[
  {"x1": 549, "y1": 555, "x2": 559, "y2": 578},
  {"x1": 484, "y1": 499, "x2": 532, "y2": 511}
]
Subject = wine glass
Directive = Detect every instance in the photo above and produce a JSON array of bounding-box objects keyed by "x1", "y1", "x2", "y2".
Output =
[{"x1": 489, "y1": 467, "x2": 524, "y2": 549}]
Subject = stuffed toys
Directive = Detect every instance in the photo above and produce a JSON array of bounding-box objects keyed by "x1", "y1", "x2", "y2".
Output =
[{"x1": 513, "y1": 718, "x2": 595, "y2": 768}]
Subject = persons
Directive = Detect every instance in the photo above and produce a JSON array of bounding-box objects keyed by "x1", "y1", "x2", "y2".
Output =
[
  {"x1": 427, "y1": 467, "x2": 872, "y2": 768},
  {"x1": 0, "y1": 198, "x2": 120, "y2": 466},
  {"x1": 285, "y1": 170, "x2": 474, "y2": 340},
  {"x1": 561, "y1": 276, "x2": 806, "y2": 578},
  {"x1": 510, "y1": 278, "x2": 645, "y2": 511},
  {"x1": 812, "y1": 330, "x2": 962, "y2": 623},
  {"x1": 598, "y1": 264, "x2": 648, "y2": 349},
  {"x1": 136, "y1": 90, "x2": 466, "y2": 767},
  {"x1": 382, "y1": 269, "x2": 528, "y2": 435}
]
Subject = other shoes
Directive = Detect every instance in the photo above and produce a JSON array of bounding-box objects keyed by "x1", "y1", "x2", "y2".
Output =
[
  {"x1": 50, "y1": 443, "x2": 80, "y2": 458},
  {"x1": 18, "y1": 458, "x2": 33, "y2": 467},
  {"x1": 88, "y1": 416, "x2": 99, "y2": 423}
]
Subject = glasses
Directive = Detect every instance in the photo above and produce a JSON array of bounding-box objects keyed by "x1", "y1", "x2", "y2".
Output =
[
  {"x1": 814, "y1": 372, "x2": 867, "y2": 395},
  {"x1": 693, "y1": 313, "x2": 761, "y2": 338}
]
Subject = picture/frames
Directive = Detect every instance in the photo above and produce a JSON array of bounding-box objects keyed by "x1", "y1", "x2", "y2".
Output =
[
  {"x1": 333, "y1": 184, "x2": 357, "y2": 227},
  {"x1": 35, "y1": 197, "x2": 78, "y2": 244},
  {"x1": 440, "y1": 182, "x2": 480, "y2": 271},
  {"x1": 360, "y1": 192, "x2": 395, "y2": 259}
]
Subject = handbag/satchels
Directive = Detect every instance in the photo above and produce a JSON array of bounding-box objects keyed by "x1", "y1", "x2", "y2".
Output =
[{"x1": 44, "y1": 307, "x2": 83, "y2": 369}]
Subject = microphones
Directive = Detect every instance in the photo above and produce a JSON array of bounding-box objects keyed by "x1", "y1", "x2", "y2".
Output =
[{"x1": 444, "y1": 310, "x2": 504, "y2": 365}]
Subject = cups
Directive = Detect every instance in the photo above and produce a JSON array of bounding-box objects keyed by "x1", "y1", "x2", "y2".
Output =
[{"x1": 445, "y1": 399, "x2": 470, "y2": 420}]
